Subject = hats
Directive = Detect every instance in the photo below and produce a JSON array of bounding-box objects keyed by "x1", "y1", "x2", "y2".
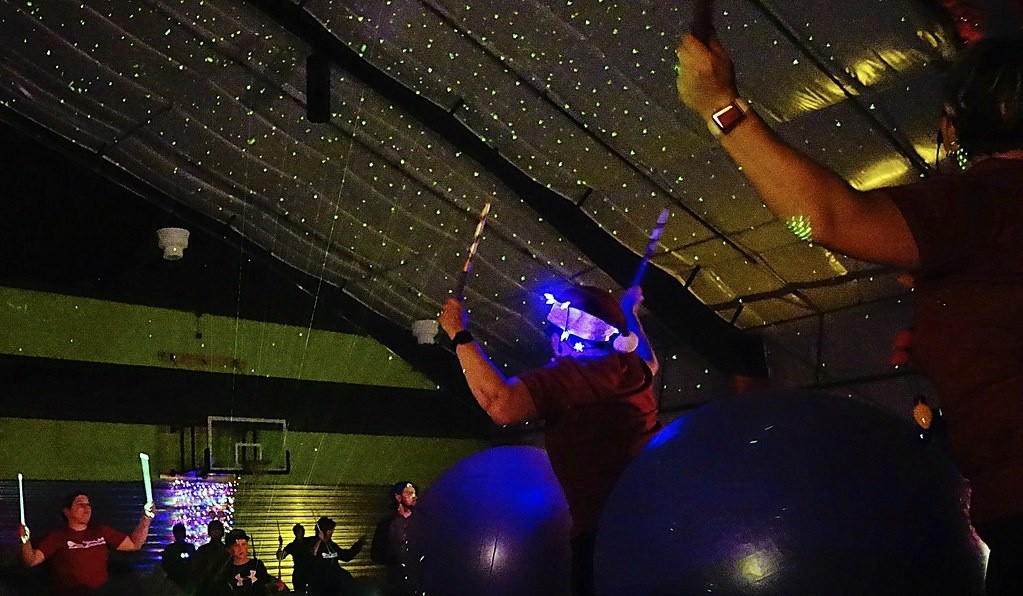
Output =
[
  {"x1": 941, "y1": 37, "x2": 1023, "y2": 132},
  {"x1": 224, "y1": 529, "x2": 250, "y2": 548},
  {"x1": 547, "y1": 287, "x2": 638, "y2": 352}
]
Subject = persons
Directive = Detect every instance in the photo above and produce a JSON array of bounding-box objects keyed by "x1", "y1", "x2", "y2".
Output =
[
  {"x1": 302, "y1": 517, "x2": 365, "y2": 596},
  {"x1": 370, "y1": 481, "x2": 427, "y2": 596},
  {"x1": 677, "y1": 29, "x2": 1017, "y2": 595},
  {"x1": 221, "y1": 529, "x2": 284, "y2": 596},
  {"x1": 186, "y1": 521, "x2": 231, "y2": 596},
  {"x1": 276, "y1": 524, "x2": 316, "y2": 596},
  {"x1": 162, "y1": 522, "x2": 197, "y2": 590},
  {"x1": 16, "y1": 491, "x2": 159, "y2": 595},
  {"x1": 442, "y1": 288, "x2": 660, "y2": 596}
]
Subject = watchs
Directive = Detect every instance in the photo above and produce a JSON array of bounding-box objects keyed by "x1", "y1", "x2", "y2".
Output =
[
  {"x1": 450, "y1": 329, "x2": 475, "y2": 354},
  {"x1": 707, "y1": 97, "x2": 753, "y2": 140}
]
